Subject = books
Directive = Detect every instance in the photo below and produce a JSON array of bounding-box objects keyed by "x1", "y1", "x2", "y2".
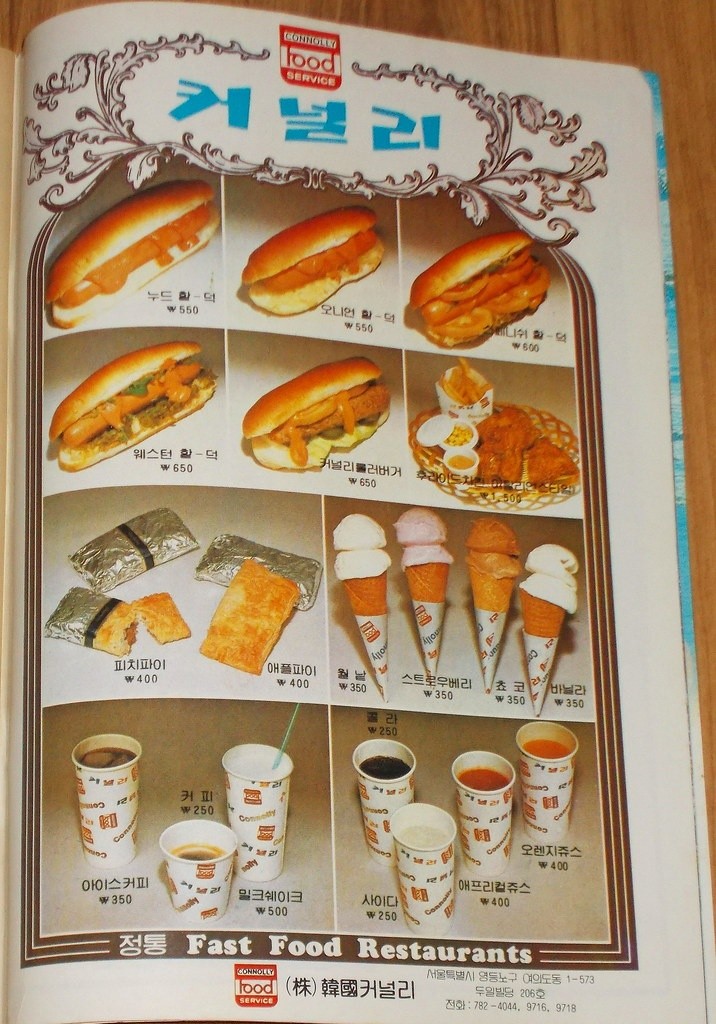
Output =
[{"x1": 0, "y1": 1, "x2": 716, "y2": 1022}]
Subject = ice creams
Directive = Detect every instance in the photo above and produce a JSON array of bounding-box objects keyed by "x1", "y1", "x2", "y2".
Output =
[{"x1": 330, "y1": 508, "x2": 578, "y2": 718}]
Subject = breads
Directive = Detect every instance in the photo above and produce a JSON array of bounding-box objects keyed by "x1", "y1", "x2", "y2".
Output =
[
  {"x1": 90, "y1": 592, "x2": 193, "y2": 658},
  {"x1": 198, "y1": 557, "x2": 300, "y2": 675}
]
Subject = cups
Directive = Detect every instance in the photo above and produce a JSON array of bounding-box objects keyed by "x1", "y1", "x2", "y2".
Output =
[
  {"x1": 443, "y1": 446, "x2": 479, "y2": 483},
  {"x1": 391, "y1": 803, "x2": 457, "y2": 939},
  {"x1": 160, "y1": 818, "x2": 238, "y2": 924},
  {"x1": 353, "y1": 738, "x2": 417, "y2": 868},
  {"x1": 452, "y1": 750, "x2": 516, "y2": 876},
  {"x1": 222, "y1": 743, "x2": 294, "y2": 883},
  {"x1": 515, "y1": 721, "x2": 578, "y2": 844},
  {"x1": 72, "y1": 732, "x2": 143, "y2": 869},
  {"x1": 437, "y1": 419, "x2": 478, "y2": 451}
]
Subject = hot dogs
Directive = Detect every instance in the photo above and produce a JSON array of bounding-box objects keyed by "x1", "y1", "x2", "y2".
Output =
[
  {"x1": 242, "y1": 203, "x2": 385, "y2": 316},
  {"x1": 242, "y1": 355, "x2": 390, "y2": 470},
  {"x1": 44, "y1": 180, "x2": 222, "y2": 325},
  {"x1": 409, "y1": 229, "x2": 551, "y2": 348},
  {"x1": 48, "y1": 340, "x2": 220, "y2": 471}
]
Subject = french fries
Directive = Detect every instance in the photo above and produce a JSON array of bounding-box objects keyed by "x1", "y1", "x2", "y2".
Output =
[{"x1": 439, "y1": 356, "x2": 494, "y2": 406}]
